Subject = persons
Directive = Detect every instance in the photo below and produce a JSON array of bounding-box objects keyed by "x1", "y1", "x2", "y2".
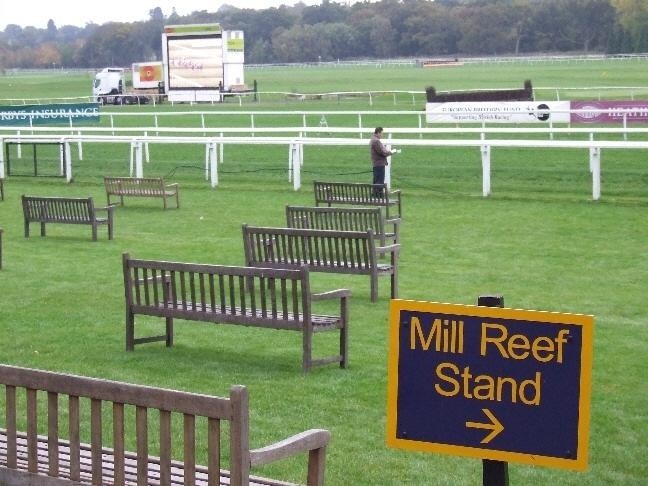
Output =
[{"x1": 368, "y1": 126, "x2": 397, "y2": 197}]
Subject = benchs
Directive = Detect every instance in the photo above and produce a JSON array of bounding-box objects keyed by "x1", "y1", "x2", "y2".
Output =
[
  {"x1": 0, "y1": 361, "x2": 332, "y2": 486},
  {"x1": 121, "y1": 249, "x2": 353, "y2": 370},
  {"x1": 21, "y1": 176, "x2": 180, "y2": 241},
  {"x1": 312, "y1": 181, "x2": 399, "y2": 220},
  {"x1": 240, "y1": 221, "x2": 401, "y2": 302},
  {"x1": 281, "y1": 202, "x2": 399, "y2": 259}
]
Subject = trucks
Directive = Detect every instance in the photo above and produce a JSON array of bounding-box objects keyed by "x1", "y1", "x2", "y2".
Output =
[{"x1": 92, "y1": 67, "x2": 168, "y2": 105}]
